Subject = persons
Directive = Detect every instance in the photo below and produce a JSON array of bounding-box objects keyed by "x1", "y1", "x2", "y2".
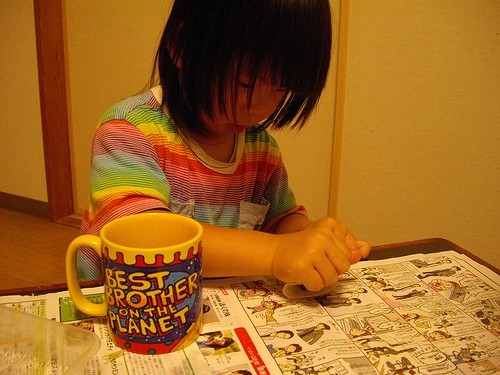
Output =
[
  {"x1": 195, "y1": 336, "x2": 234, "y2": 357},
  {"x1": 212, "y1": 369, "x2": 252, "y2": 375},
  {"x1": 76, "y1": 0, "x2": 371, "y2": 292}
]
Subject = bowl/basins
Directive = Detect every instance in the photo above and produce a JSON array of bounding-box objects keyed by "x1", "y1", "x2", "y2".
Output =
[{"x1": 0, "y1": 303, "x2": 101, "y2": 374}]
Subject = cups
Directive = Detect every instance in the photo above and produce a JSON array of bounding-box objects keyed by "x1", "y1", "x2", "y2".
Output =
[{"x1": 65, "y1": 212, "x2": 202, "y2": 354}]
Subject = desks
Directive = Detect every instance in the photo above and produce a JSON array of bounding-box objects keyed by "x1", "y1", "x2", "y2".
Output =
[{"x1": 0, "y1": 237, "x2": 500, "y2": 375}]
